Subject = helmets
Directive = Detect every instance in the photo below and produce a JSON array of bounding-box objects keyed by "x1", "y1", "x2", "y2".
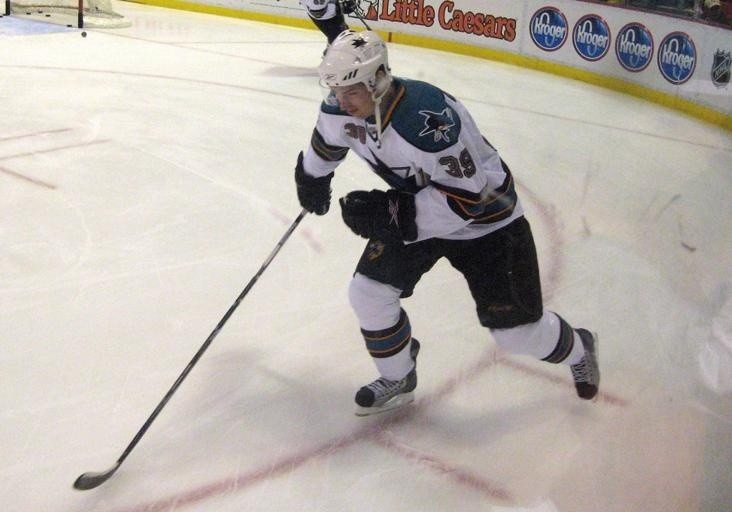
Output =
[{"x1": 320, "y1": 27, "x2": 392, "y2": 95}]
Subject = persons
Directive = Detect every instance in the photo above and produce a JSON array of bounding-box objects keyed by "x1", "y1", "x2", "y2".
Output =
[
  {"x1": 302, "y1": 0, "x2": 359, "y2": 56},
  {"x1": 295, "y1": 30, "x2": 601, "y2": 409}
]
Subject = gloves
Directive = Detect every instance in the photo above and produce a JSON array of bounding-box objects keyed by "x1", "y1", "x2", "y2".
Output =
[
  {"x1": 294, "y1": 150, "x2": 334, "y2": 215},
  {"x1": 339, "y1": 189, "x2": 417, "y2": 243}
]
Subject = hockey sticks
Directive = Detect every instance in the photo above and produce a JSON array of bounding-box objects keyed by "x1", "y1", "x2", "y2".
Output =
[{"x1": 74, "y1": 188, "x2": 334, "y2": 491}]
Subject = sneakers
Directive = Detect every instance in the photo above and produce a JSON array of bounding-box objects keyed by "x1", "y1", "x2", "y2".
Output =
[
  {"x1": 568, "y1": 328, "x2": 600, "y2": 400},
  {"x1": 355, "y1": 366, "x2": 417, "y2": 408}
]
